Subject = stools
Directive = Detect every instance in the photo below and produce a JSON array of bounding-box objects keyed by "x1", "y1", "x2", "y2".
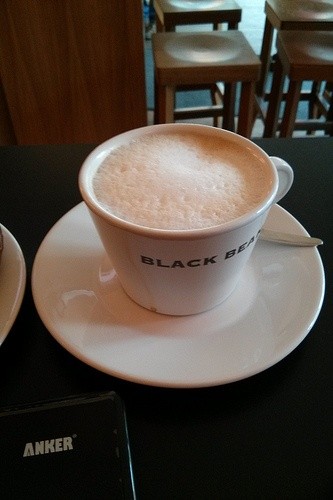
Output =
[
  {"x1": 257, "y1": 0, "x2": 333, "y2": 139},
  {"x1": 152, "y1": 0, "x2": 261, "y2": 138}
]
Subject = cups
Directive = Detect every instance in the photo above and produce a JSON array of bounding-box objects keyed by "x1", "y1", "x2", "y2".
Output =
[{"x1": 77, "y1": 122, "x2": 296, "y2": 316}]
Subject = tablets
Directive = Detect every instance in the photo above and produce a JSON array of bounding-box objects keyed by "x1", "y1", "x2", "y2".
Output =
[{"x1": 0, "y1": 387, "x2": 137, "y2": 500}]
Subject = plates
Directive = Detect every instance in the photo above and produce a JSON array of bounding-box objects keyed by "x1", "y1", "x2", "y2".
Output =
[
  {"x1": 0, "y1": 222, "x2": 26, "y2": 347},
  {"x1": 31, "y1": 199, "x2": 326, "y2": 388}
]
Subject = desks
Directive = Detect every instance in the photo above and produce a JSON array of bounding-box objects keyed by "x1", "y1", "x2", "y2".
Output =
[{"x1": 0, "y1": 137, "x2": 333, "y2": 500}]
